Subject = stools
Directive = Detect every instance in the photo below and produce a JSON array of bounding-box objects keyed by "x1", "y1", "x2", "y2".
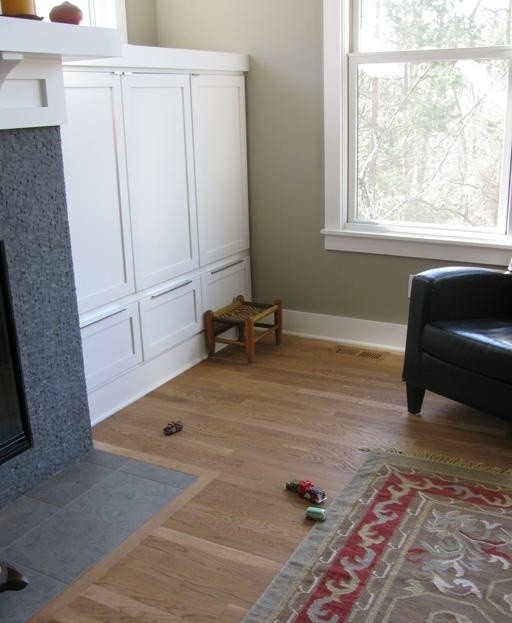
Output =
[{"x1": 206, "y1": 296, "x2": 283, "y2": 363}]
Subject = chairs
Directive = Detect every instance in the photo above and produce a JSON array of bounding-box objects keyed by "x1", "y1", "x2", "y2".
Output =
[{"x1": 402, "y1": 262, "x2": 512, "y2": 415}]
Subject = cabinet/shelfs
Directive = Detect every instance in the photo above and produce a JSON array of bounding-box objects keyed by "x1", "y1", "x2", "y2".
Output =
[
  {"x1": 190, "y1": 47, "x2": 255, "y2": 362},
  {"x1": 58, "y1": 43, "x2": 203, "y2": 428}
]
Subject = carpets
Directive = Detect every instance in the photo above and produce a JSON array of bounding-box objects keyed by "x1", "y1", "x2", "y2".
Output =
[{"x1": 241, "y1": 446, "x2": 511, "y2": 623}]
